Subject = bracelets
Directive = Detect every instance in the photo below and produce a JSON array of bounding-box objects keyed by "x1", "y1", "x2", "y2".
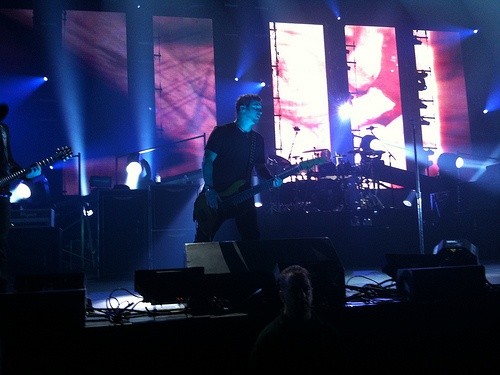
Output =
[{"x1": 204, "y1": 184, "x2": 217, "y2": 193}]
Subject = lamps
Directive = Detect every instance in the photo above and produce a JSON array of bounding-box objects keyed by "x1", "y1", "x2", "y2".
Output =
[
  {"x1": 21, "y1": 172, "x2": 63, "y2": 198},
  {"x1": 127, "y1": 153, "x2": 151, "y2": 183},
  {"x1": 437, "y1": 153, "x2": 464, "y2": 169}
]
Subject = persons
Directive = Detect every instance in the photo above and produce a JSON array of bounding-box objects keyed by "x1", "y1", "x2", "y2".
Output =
[
  {"x1": 251, "y1": 265, "x2": 336, "y2": 375},
  {"x1": 185, "y1": 94, "x2": 282, "y2": 309},
  {"x1": 0, "y1": 103, "x2": 42, "y2": 244}
]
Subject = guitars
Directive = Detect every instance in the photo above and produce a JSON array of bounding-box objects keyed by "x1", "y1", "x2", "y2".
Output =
[
  {"x1": 196, "y1": 152, "x2": 331, "y2": 223},
  {"x1": 0, "y1": 145, "x2": 74, "y2": 202}
]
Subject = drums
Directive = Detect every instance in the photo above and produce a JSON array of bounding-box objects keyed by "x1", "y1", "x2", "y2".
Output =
[{"x1": 312, "y1": 161, "x2": 361, "y2": 209}]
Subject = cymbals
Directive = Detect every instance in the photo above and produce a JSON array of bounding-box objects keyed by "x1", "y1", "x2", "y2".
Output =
[{"x1": 303, "y1": 149, "x2": 328, "y2": 152}]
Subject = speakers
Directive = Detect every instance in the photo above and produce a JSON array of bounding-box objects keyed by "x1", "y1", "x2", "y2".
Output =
[
  {"x1": 148, "y1": 186, "x2": 197, "y2": 270},
  {"x1": 395, "y1": 264, "x2": 486, "y2": 302},
  {"x1": 133, "y1": 267, "x2": 203, "y2": 307},
  {"x1": 0, "y1": 288, "x2": 85, "y2": 332},
  {"x1": 99, "y1": 193, "x2": 149, "y2": 281},
  {"x1": 184, "y1": 241, "x2": 257, "y2": 314},
  {"x1": 248, "y1": 237, "x2": 346, "y2": 308},
  {"x1": 7, "y1": 227, "x2": 62, "y2": 265}
]
